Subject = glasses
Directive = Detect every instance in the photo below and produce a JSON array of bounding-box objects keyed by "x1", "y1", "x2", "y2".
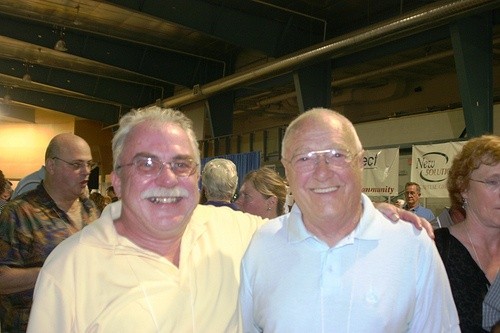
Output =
[
  {"x1": 466, "y1": 177, "x2": 500, "y2": 191},
  {"x1": 115, "y1": 156, "x2": 199, "y2": 176},
  {"x1": 283, "y1": 149, "x2": 365, "y2": 168},
  {"x1": 51, "y1": 156, "x2": 97, "y2": 170}
]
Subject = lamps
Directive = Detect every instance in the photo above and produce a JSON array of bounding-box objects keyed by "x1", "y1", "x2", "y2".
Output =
[{"x1": 53, "y1": 27, "x2": 69, "y2": 52}]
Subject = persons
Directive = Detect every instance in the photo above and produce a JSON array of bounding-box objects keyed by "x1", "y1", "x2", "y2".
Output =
[
  {"x1": 202, "y1": 158, "x2": 241, "y2": 209},
  {"x1": 236, "y1": 167, "x2": 287, "y2": 221},
  {"x1": 0, "y1": 133, "x2": 101, "y2": 333},
  {"x1": 26, "y1": 106, "x2": 436, "y2": 333},
  {"x1": 432, "y1": 134, "x2": 500, "y2": 333},
  {"x1": 403, "y1": 182, "x2": 435, "y2": 223},
  {"x1": 0, "y1": 165, "x2": 121, "y2": 220},
  {"x1": 239, "y1": 107, "x2": 461, "y2": 333}
]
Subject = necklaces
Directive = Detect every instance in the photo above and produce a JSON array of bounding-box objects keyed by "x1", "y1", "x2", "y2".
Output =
[{"x1": 463, "y1": 219, "x2": 500, "y2": 290}]
